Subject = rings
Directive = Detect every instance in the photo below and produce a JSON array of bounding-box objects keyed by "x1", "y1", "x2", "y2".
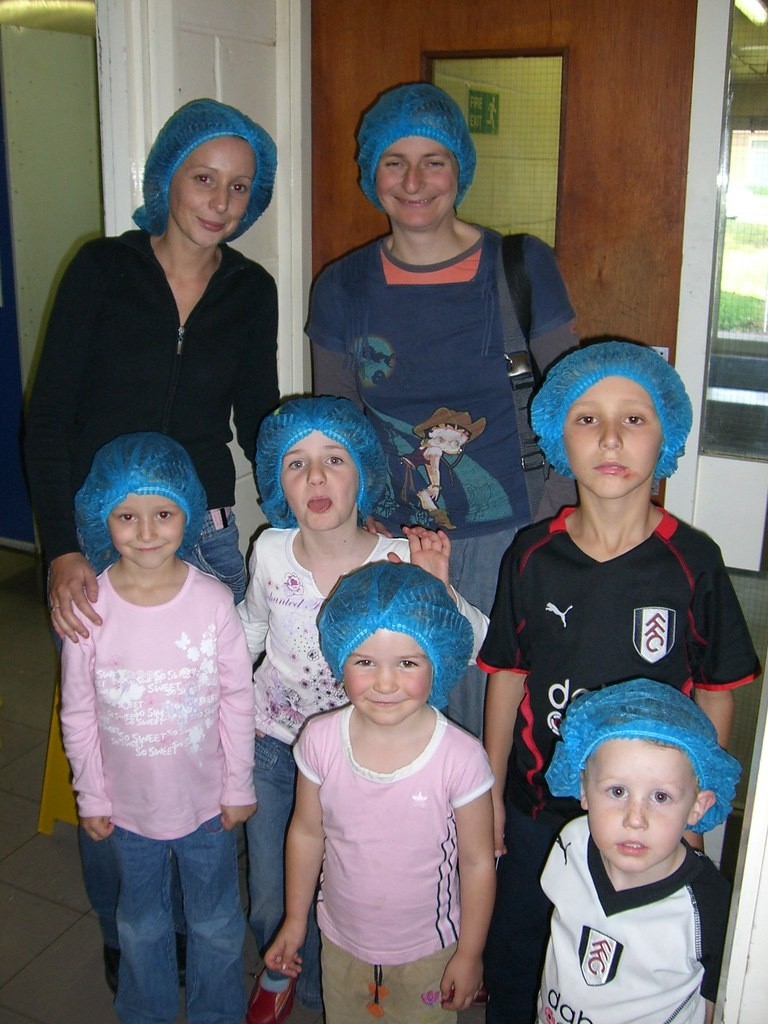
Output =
[{"x1": 50, "y1": 606, "x2": 59, "y2": 612}]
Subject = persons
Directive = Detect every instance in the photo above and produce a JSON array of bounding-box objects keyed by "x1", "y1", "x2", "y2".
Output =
[
  {"x1": 535, "y1": 678, "x2": 744, "y2": 1024},
  {"x1": 59, "y1": 432, "x2": 259, "y2": 1024},
  {"x1": 263, "y1": 568, "x2": 497, "y2": 1024},
  {"x1": 23, "y1": 97, "x2": 394, "y2": 1024},
  {"x1": 477, "y1": 341, "x2": 761, "y2": 1024},
  {"x1": 304, "y1": 80, "x2": 581, "y2": 1024},
  {"x1": 234, "y1": 394, "x2": 490, "y2": 1023}
]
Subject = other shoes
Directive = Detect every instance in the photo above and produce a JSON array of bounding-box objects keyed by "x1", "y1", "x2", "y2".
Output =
[
  {"x1": 246, "y1": 967, "x2": 295, "y2": 1024},
  {"x1": 105, "y1": 959, "x2": 119, "y2": 993},
  {"x1": 177, "y1": 946, "x2": 187, "y2": 985}
]
